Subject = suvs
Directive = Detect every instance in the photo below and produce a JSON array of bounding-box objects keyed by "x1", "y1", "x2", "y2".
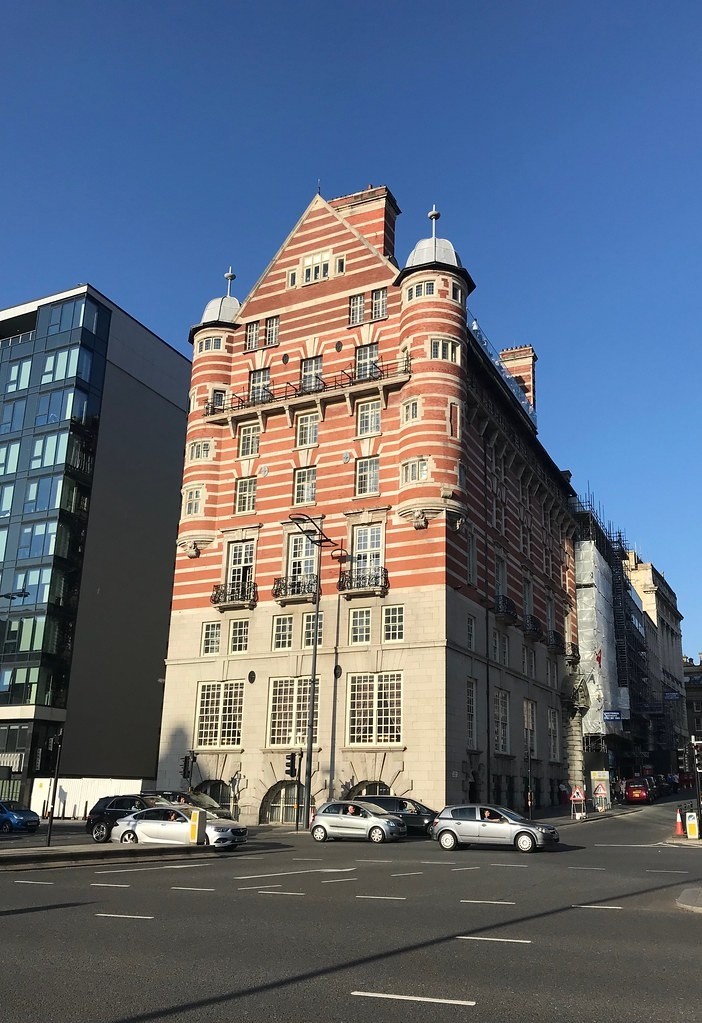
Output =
[{"x1": 86, "y1": 794, "x2": 175, "y2": 843}]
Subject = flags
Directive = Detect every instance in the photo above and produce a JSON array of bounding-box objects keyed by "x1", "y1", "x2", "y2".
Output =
[{"x1": 596, "y1": 649, "x2": 601, "y2": 668}]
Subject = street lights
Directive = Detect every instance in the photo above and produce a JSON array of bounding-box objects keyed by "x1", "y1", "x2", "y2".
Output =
[{"x1": 288, "y1": 512, "x2": 338, "y2": 832}]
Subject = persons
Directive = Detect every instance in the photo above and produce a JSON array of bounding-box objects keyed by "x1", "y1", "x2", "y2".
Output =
[
  {"x1": 167, "y1": 812, "x2": 178, "y2": 821},
  {"x1": 131, "y1": 800, "x2": 142, "y2": 811},
  {"x1": 179, "y1": 796, "x2": 187, "y2": 803},
  {"x1": 399, "y1": 802, "x2": 414, "y2": 813},
  {"x1": 481, "y1": 809, "x2": 500, "y2": 822},
  {"x1": 346, "y1": 805, "x2": 356, "y2": 815},
  {"x1": 610, "y1": 771, "x2": 683, "y2": 804}
]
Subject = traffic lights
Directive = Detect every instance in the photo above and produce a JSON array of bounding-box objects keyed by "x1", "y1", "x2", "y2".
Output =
[
  {"x1": 179, "y1": 756, "x2": 190, "y2": 779},
  {"x1": 285, "y1": 753, "x2": 296, "y2": 777},
  {"x1": 678, "y1": 742, "x2": 695, "y2": 773},
  {"x1": 694, "y1": 741, "x2": 702, "y2": 772}
]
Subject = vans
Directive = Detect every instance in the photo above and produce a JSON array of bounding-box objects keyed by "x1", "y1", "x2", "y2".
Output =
[
  {"x1": 139, "y1": 790, "x2": 231, "y2": 820},
  {"x1": 352, "y1": 796, "x2": 439, "y2": 837}
]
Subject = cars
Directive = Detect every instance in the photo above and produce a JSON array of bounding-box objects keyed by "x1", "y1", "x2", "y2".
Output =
[
  {"x1": 433, "y1": 805, "x2": 560, "y2": 852},
  {"x1": 0, "y1": 800, "x2": 40, "y2": 834},
  {"x1": 112, "y1": 804, "x2": 248, "y2": 851},
  {"x1": 311, "y1": 801, "x2": 408, "y2": 844},
  {"x1": 626, "y1": 774, "x2": 675, "y2": 803}
]
